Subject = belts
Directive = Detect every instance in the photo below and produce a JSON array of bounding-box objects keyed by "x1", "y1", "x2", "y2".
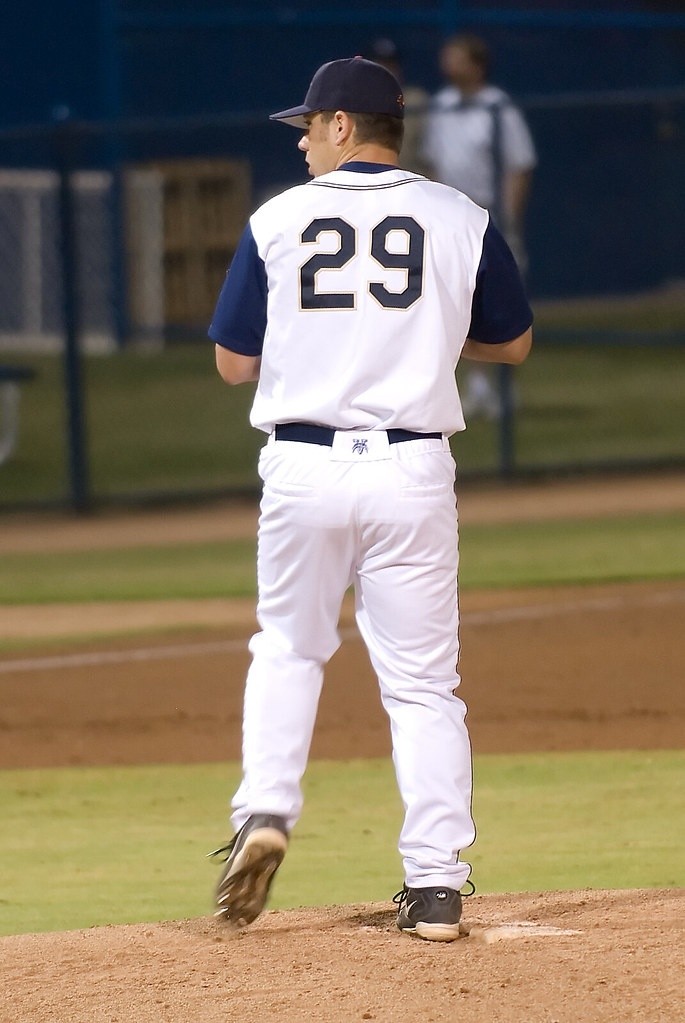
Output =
[{"x1": 276, "y1": 422, "x2": 442, "y2": 447}]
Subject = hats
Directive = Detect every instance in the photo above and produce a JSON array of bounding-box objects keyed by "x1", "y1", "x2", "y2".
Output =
[{"x1": 269, "y1": 55, "x2": 405, "y2": 129}]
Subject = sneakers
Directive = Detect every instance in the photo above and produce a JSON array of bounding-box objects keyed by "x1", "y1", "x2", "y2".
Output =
[
  {"x1": 392, "y1": 880, "x2": 476, "y2": 942},
  {"x1": 206, "y1": 814, "x2": 289, "y2": 926}
]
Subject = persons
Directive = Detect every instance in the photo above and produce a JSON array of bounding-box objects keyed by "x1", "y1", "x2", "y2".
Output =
[
  {"x1": 361, "y1": 38, "x2": 432, "y2": 171},
  {"x1": 208, "y1": 54, "x2": 535, "y2": 940},
  {"x1": 418, "y1": 36, "x2": 542, "y2": 416}
]
